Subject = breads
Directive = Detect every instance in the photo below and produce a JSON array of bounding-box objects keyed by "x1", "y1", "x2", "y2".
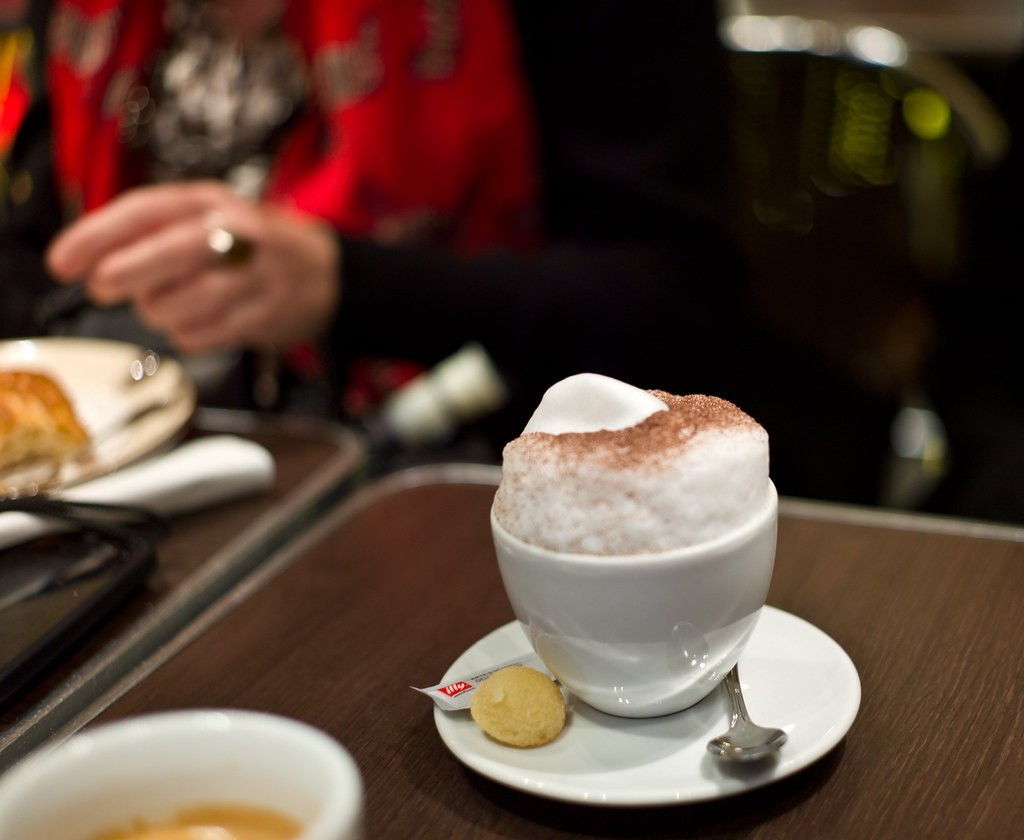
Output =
[
  {"x1": 0, "y1": 369, "x2": 92, "y2": 480},
  {"x1": 467, "y1": 666, "x2": 565, "y2": 746}
]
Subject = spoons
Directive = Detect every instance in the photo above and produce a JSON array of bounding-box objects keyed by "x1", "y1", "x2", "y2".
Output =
[{"x1": 706, "y1": 663, "x2": 787, "y2": 762}]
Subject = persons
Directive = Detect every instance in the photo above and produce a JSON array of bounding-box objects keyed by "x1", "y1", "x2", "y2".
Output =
[{"x1": 1, "y1": 0, "x2": 731, "y2": 474}]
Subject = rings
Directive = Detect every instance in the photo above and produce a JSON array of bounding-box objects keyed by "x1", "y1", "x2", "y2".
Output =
[{"x1": 211, "y1": 228, "x2": 249, "y2": 262}]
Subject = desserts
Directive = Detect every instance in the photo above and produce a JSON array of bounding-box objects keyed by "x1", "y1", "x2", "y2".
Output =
[{"x1": 492, "y1": 373, "x2": 768, "y2": 560}]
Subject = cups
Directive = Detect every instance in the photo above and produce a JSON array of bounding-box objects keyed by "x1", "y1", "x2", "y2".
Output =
[{"x1": 490, "y1": 474, "x2": 779, "y2": 717}]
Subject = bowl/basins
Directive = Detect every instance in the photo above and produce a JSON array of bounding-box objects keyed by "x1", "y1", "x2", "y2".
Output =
[{"x1": 0, "y1": 707, "x2": 366, "y2": 840}]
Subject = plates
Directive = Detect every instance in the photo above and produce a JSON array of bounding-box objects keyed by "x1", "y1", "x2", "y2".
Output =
[
  {"x1": 434, "y1": 604, "x2": 863, "y2": 805},
  {"x1": 0, "y1": 337, "x2": 194, "y2": 501}
]
